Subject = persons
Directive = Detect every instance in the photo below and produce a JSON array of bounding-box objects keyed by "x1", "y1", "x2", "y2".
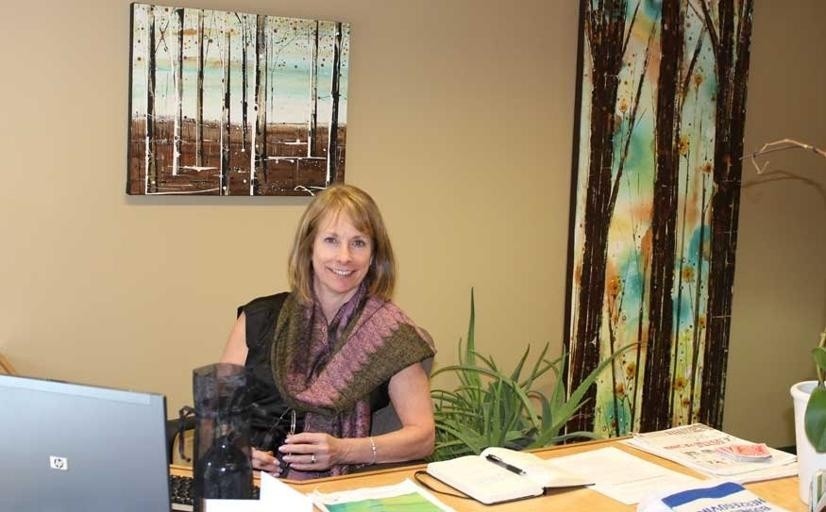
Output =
[{"x1": 197, "y1": 183, "x2": 438, "y2": 484}]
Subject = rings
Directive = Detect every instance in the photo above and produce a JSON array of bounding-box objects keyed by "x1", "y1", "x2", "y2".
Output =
[{"x1": 311, "y1": 454, "x2": 316, "y2": 464}]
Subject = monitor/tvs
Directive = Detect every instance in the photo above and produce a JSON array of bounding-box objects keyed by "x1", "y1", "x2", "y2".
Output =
[{"x1": 0, "y1": 374, "x2": 173, "y2": 512}]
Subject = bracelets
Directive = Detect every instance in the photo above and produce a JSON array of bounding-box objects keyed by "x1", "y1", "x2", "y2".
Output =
[{"x1": 367, "y1": 434, "x2": 377, "y2": 466}]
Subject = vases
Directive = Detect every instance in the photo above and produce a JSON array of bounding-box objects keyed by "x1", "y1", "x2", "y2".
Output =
[{"x1": 790, "y1": 380, "x2": 825, "y2": 499}]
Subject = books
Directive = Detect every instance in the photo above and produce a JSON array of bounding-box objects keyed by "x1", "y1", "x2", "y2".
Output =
[{"x1": 424, "y1": 445, "x2": 596, "y2": 506}]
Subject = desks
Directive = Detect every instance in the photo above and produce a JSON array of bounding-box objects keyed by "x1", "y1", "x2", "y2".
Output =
[{"x1": 172, "y1": 422, "x2": 826, "y2": 512}]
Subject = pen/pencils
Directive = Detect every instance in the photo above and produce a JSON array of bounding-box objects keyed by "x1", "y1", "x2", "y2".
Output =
[{"x1": 485, "y1": 454, "x2": 527, "y2": 475}]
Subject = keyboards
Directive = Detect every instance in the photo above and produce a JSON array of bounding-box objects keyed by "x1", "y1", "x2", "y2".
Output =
[{"x1": 171, "y1": 474, "x2": 260, "y2": 512}]
